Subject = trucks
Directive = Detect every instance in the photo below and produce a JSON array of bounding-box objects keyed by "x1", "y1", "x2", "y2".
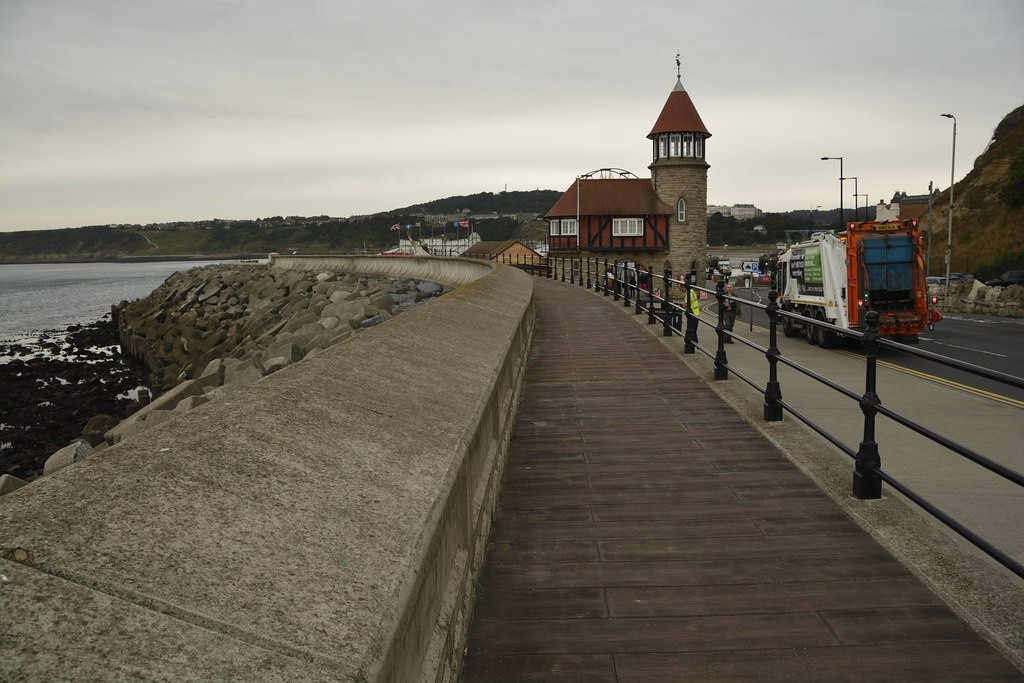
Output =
[{"x1": 770, "y1": 218, "x2": 943, "y2": 347}]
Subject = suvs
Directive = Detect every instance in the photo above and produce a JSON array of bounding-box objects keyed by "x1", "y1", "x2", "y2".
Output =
[
  {"x1": 924, "y1": 276, "x2": 958, "y2": 304},
  {"x1": 944, "y1": 272, "x2": 975, "y2": 279},
  {"x1": 986, "y1": 271, "x2": 1024, "y2": 287}
]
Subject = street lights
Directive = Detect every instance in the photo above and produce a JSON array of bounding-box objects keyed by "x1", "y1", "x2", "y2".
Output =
[
  {"x1": 853, "y1": 194, "x2": 868, "y2": 222},
  {"x1": 839, "y1": 177, "x2": 857, "y2": 222},
  {"x1": 940, "y1": 114, "x2": 956, "y2": 289},
  {"x1": 821, "y1": 157, "x2": 843, "y2": 231}
]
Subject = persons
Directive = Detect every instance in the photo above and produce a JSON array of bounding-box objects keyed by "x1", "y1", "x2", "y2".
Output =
[
  {"x1": 723, "y1": 284, "x2": 742, "y2": 344},
  {"x1": 684, "y1": 284, "x2": 699, "y2": 343},
  {"x1": 629, "y1": 276, "x2": 636, "y2": 297}
]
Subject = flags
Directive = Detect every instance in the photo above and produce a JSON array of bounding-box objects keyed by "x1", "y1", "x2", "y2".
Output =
[
  {"x1": 454, "y1": 221, "x2": 458, "y2": 227},
  {"x1": 459, "y1": 220, "x2": 469, "y2": 227},
  {"x1": 391, "y1": 224, "x2": 400, "y2": 231},
  {"x1": 404, "y1": 223, "x2": 412, "y2": 229},
  {"x1": 415, "y1": 222, "x2": 420, "y2": 227}
]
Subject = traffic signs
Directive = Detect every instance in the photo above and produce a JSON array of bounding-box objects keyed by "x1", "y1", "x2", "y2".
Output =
[{"x1": 740, "y1": 261, "x2": 758, "y2": 272}]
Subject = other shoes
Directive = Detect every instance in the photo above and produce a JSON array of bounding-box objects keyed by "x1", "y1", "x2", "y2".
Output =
[{"x1": 728, "y1": 339, "x2": 734, "y2": 344}]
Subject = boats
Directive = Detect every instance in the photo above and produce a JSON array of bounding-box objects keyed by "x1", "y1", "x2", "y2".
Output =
[
  {"x1": 718, "y1": 260, "x2": 732, "y2": 276},
  {"x1": 240, "y1": 260, "x2": 258, "y2": 263}
]
